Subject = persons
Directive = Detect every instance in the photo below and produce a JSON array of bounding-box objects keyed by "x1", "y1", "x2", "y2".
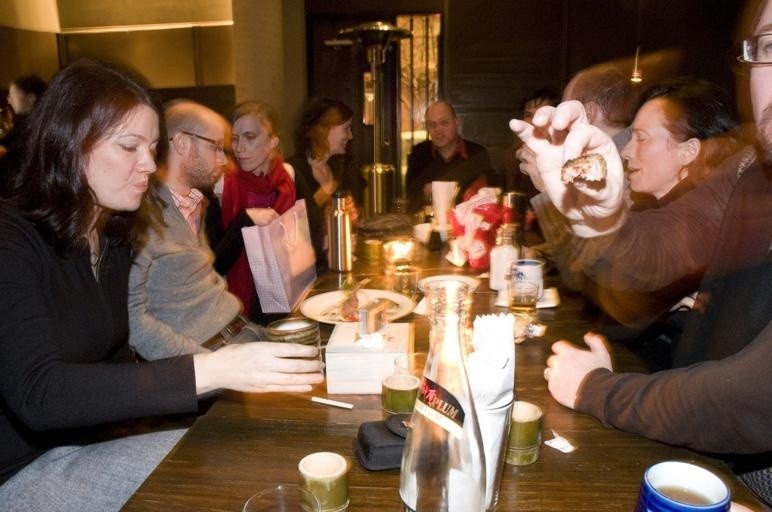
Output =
[{"x1": 405, "y1": 100, "x2": 496, "y2": 216}]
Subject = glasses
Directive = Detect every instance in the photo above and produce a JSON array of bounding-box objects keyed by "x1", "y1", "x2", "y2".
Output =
[
  {"x1": 736, "y1": 32, "x2": 772, "y2": 66},
  {"x1": 169, "y1": 130, "x2": 225, "y2": 153}
]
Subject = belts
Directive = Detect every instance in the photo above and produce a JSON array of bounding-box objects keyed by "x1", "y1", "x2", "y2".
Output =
[{"x1": 198, "y1": 313, "x2": 251, "y2": 349}]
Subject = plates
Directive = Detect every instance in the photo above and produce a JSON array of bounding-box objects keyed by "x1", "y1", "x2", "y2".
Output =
[
  {"x1": 413, "y1": 223, "x2": 453, "y2": 244},
  {"x1": 299, "y1": 288, "x2": 415, "y2": 326}
]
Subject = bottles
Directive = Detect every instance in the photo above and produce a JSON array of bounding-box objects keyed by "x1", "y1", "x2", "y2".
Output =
[
  {"x1": 428, "y1": 224, "x2": 443, "y2": 251},
  {"x1": 398, "y1": 275, "x2": 486, "y2": 510},
  {"x1": 329, "y1": 192, "x2": 352, "y2": 274},
  {"x1": 490, "y1": 192, "x2": 522, "y2": 292}
]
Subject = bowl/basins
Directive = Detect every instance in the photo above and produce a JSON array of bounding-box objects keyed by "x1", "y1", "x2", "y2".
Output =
[{"x1": 417, "y1": 274, "x2": 481, "y2": 296}]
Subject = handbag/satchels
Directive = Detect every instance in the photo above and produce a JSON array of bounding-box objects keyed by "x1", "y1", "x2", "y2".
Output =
[
  {"x1": 352, "y1": 410, "x2": 415, "y2": 475},
  {"x1": 239, "y1": 197, "x2": 318, "y2": 314}
]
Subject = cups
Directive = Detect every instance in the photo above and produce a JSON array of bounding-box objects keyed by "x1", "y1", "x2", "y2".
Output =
[
  {"x1": 391, "y1": 271, "x2": 416, "y2": 298},
  {"x1": 266, "y1": 316, "x2": 324, "y2": 380},
  {"x1": 364, "y1": 239, "x2": 383, "y2": 264},
  {"x1": 471, "y1": 389, "x2": 519, "y2": 510},
  {"x1": 382, "y1": 374, "x2": 420, "y2": 421},
  {"x1": 636, "y1": 460, "x2": 732, "y2": 512},
  {"x1": 506, "y1": 401, "x2": 544, "y2": 467},
  {"x1": 243, "y1": 482, "x2": 322, "y2": 511},
  {"x1": 512, "y1": 258, "x2": 545, "y2": 298},
  {"x1": 394, "y1": 352, "x2": 433, "y2": 376},
  {"x1": 298, "y1": 450, "x2": 351, "y2": 512},
  {"x1": 508, "y1": 281, "x2": 539, "y2": 327}
]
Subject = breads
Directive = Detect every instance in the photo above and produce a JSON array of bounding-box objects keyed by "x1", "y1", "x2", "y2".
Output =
[{"x1": 561, "y1": 153, "x2": 607, "y2": 184}]
